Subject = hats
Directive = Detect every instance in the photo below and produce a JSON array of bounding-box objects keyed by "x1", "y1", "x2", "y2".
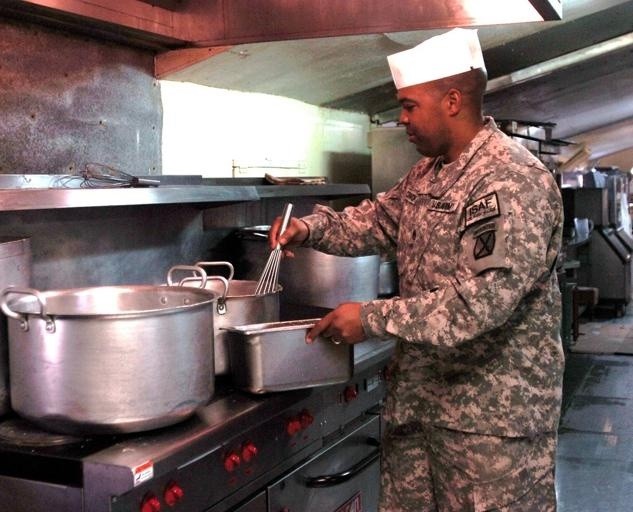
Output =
[{"x1": 387, "y1": 27, "x2": 486, "y2": 90}]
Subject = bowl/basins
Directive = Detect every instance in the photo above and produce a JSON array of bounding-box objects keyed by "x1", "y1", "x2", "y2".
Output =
[
  {"x1": 235, "y1": 219, "x2": 380, "y2": 313},
  {"x1": 378, "y1": 256, "x2": 397, "y2": 294}
]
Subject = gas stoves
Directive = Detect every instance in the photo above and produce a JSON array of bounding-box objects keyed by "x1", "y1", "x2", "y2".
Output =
[{"x1": 0, "y1": 332, "x2": 400, "y2": 511}]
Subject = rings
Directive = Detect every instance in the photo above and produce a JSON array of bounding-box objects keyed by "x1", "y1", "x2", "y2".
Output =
[{"x1": 331, "y1": 336, "x2": 341, "y2": 344}]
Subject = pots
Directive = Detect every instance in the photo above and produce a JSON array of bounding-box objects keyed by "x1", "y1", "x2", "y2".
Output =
[
  {"x1": 162, "y1": 258, "x2": 282, "y2": 377},
  {"x1": 0, "y1": 264, "x2": 219, "y2": 438}
]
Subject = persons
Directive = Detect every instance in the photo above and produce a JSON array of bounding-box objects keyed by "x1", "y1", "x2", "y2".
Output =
[{"x1": 269, "y1": 26, "x2": 566, "y2": 512}]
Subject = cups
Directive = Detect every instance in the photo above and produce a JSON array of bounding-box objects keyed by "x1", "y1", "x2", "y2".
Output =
[{"x1": 573, "y1": 217, "x2": 594, "y2": 239}]
